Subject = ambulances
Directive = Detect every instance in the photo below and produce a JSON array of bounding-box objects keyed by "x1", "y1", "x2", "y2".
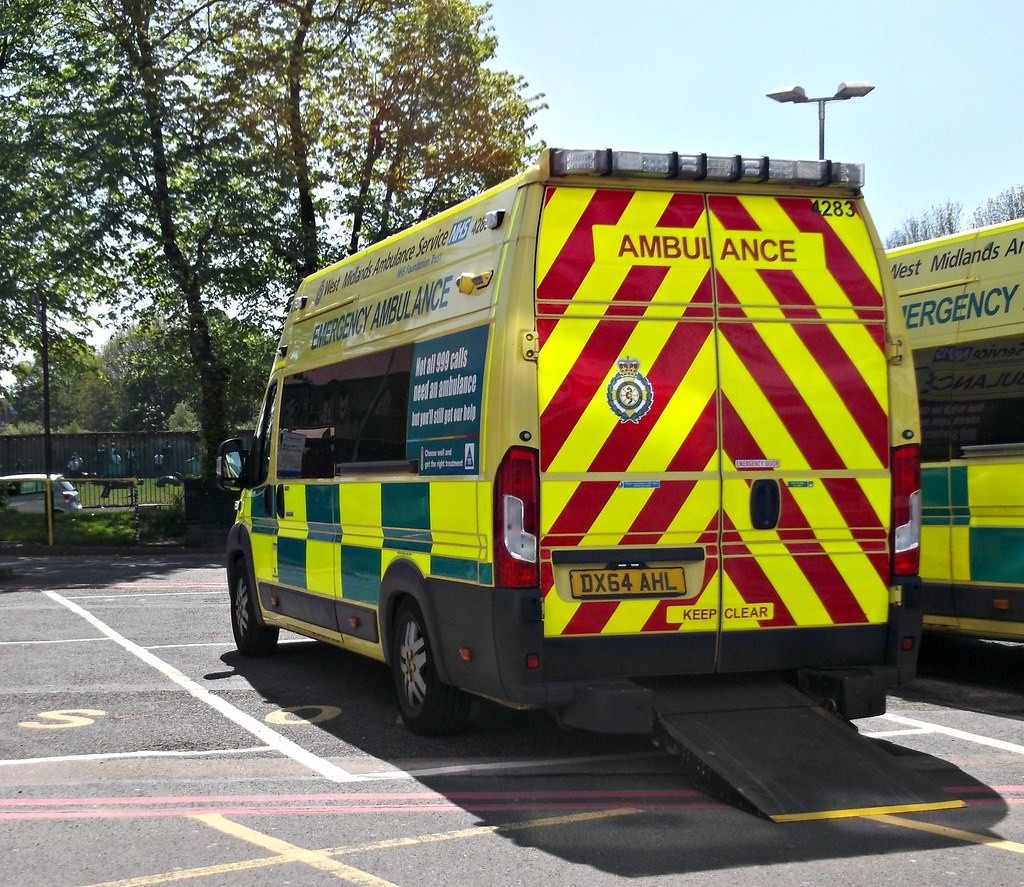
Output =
[{"x1": 213, "y1": 146, "x2": 971, "y2": 822}]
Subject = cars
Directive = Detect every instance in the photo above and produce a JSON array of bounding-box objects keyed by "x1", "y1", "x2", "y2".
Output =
[{"x1": 0, "y1": 474, "x2": 84, "y2": 517}]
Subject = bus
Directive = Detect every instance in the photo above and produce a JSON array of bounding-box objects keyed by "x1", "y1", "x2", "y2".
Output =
[{"x1": 884, "y1": 218, "x2": 1024, "y2": 640}]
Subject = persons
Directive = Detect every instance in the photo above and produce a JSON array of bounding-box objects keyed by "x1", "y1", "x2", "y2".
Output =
[
  {"x1": 154, "y1": 450, "x2": 163, "y2": 477},
  {"x1": 162, "y1": 440, "x2": 173, "y2": 467},
  {"x1": 14, "y1": 459, "x2": 25, "y2": 474},
  {"x1": 68, "y1": 442, "x2": 137, "y2": 478}
]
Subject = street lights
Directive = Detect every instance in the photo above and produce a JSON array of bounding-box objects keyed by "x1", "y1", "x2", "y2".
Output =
[{"x1": 764, "y1": 79, "x2": 876, "y2": 161}]
